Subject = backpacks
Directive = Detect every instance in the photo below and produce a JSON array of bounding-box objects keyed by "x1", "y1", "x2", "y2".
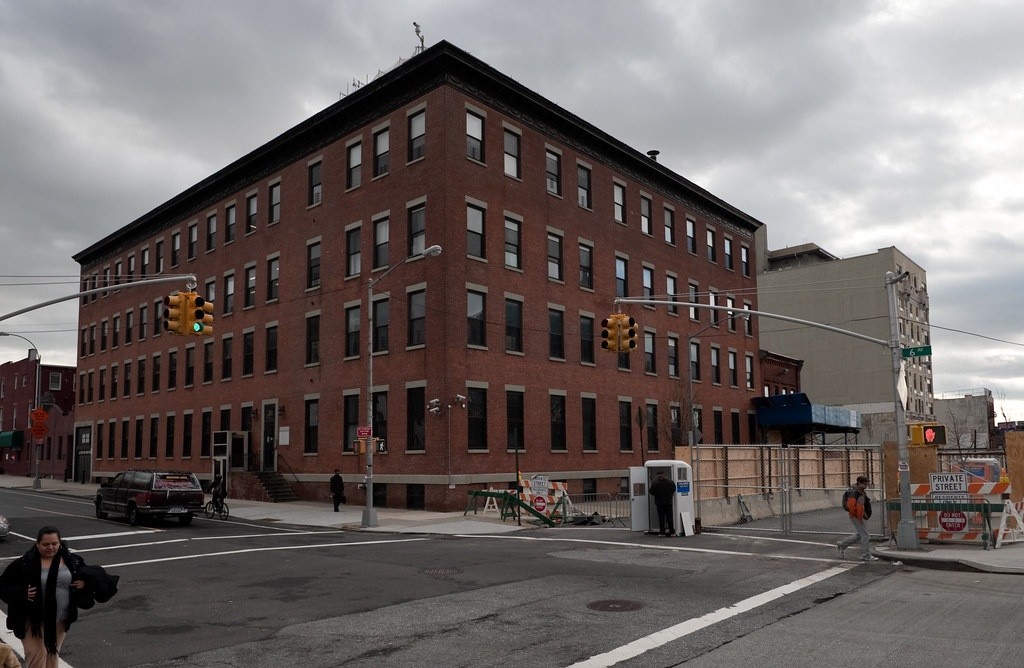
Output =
[
  {"x1": 842, "y1": 487, "x2": 853, "y2": 512},
  {"x1": 217, "y1": 484, "x2": 226, "y2": 498}
]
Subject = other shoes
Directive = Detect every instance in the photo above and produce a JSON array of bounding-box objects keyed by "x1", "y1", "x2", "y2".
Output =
[
  {"x1": 670, "y1": 533, "x2": 676, "y2": 537},
  {"x1": 862, "y1": 553, "x2": 879, "y2": 561},
  {"x1": 658, "y1": 534, "x2": 665, "y2": 537},
  {"x1": 837, "y1": 541, "x2": 844, "y2": 556}
]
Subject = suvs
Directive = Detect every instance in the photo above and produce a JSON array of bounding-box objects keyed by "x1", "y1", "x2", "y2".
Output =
[{"x1": 94, "y1": 469, "x2": 206, "y2": 527}]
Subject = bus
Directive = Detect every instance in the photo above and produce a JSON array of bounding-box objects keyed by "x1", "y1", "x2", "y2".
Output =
[{"x1": 951, "y1": 458, "x2": 1002, "y2": 483}]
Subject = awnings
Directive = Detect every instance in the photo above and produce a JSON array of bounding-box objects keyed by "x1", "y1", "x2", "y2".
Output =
[{"x1": 0, "y1": 430, "x2": 25, "y2": 448}]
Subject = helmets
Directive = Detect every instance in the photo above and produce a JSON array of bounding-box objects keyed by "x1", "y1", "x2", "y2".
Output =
[{"x1": 216, "y1": 474, "x2": 222, "y2": 479}]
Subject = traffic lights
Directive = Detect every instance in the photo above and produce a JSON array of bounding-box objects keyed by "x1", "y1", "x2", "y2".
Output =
[
  {"x1": 619, "y1": 316, "x2": 639, "y2": 352},
  {"x1": 372, "y1": 438, "x2": 386, "y2": 453},
  {"x1": 600, "y1": 317, "x2": 618, "y2": 352},
  {"x1": 922, "y1": 423, "x2": 947, "y2": 447},
  {"x1": 353, "y1": 440, "x2": 366, "y2": 454},
  {"x1": 164, "y1": 292, "x2": 185, "y2": 334},
  {"x1": 187, "y1": 293, "x2": 214, "y2": 337}
]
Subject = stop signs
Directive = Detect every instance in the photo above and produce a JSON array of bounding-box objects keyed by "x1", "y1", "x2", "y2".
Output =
[
  {"x1": 532, "y1": 494, "x2": 547, "y2": 513},
  {"x1": 937, "y1": 508, "x2": 968, "y2": 534}
]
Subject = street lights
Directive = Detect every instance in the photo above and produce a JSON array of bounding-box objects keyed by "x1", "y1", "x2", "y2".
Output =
[
  {"x1": 361, "y1": 245, "x2": 442, "y2": 527},
  {"x1": 687, "y1": 312, "x2": 750, "y2": 439},
  {"x1": 887, "y1": 270, "x2": 921, "y2": 550},
  {"x1": 0, "y1": 332, "x2": 42, "y2": 490}
]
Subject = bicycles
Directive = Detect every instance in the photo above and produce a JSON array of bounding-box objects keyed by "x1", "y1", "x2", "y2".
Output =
[{"x1": 205, "y1": 491, "x2": 229, "y2": 521}]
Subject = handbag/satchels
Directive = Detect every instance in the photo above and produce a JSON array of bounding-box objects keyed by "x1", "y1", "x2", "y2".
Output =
[{"x1": 340, "y1": 495, "x2": 346, "y2": 503}]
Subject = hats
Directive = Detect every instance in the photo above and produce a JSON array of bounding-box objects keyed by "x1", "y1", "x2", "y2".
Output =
[{"x1": 857, "y1": 477, "x2": 870, "y2": 483}]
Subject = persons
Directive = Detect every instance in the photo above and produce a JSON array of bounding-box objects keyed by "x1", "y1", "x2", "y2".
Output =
[
  {"x1": 649, "y1": 471, "x2": 676, "y2": 537},
  {"x1": 0, "y1": 526, "x2": 87, "y2": 668},
  {"x1": 330, "y1": 469, "x2": 345, "y2": 512},
  {"x1": 837, "y1": 476, "x2": 879, "y2": 561},
  {"x1": 209, "y1": 475, "x2": 225, "y2": 513}
]
